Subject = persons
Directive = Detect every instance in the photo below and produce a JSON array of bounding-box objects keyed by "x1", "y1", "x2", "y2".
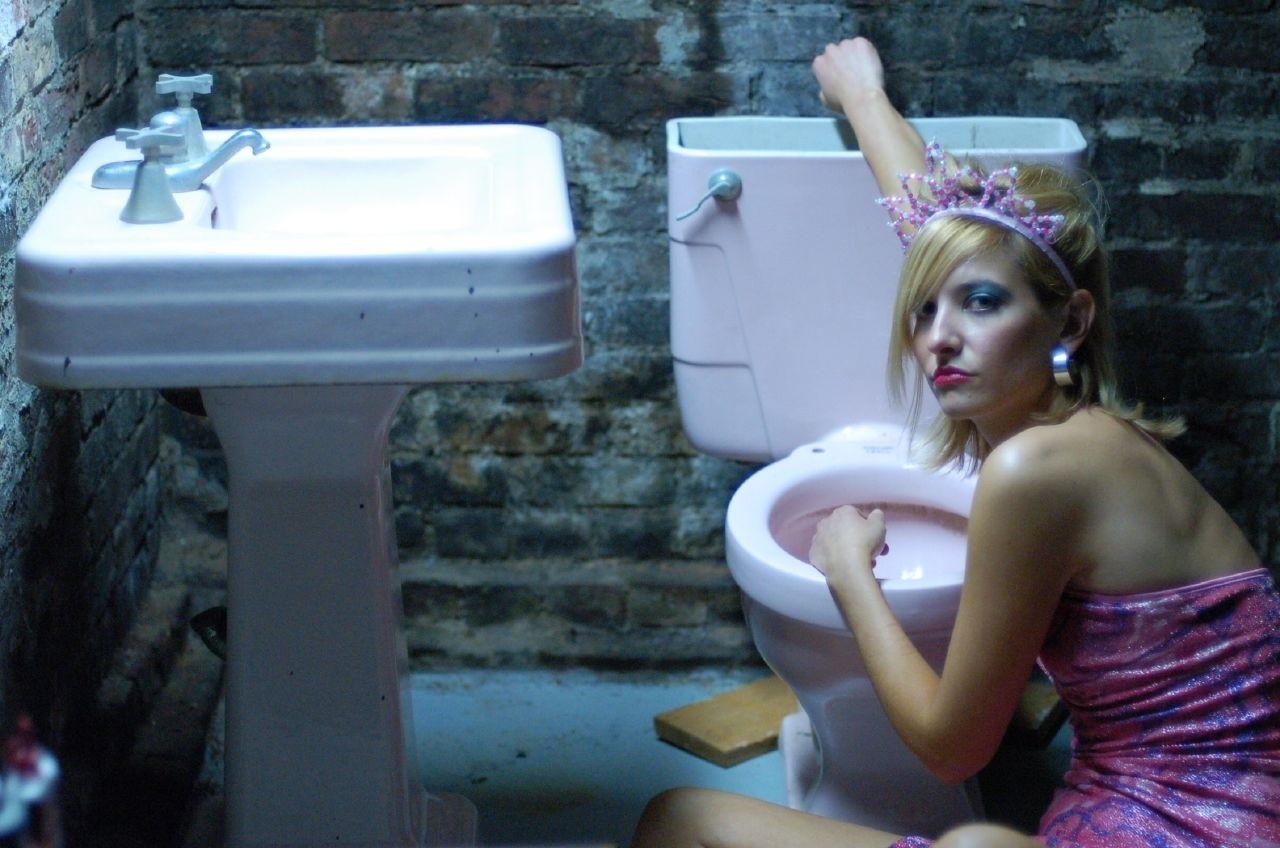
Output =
[{"x1": 629, "y1": 35, "x2": 1280, "y2": 848}]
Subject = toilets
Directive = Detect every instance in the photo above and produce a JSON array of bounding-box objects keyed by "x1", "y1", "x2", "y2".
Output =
[{"x1": 665, "y1": 112, "x2": 1090, "y2": 841}]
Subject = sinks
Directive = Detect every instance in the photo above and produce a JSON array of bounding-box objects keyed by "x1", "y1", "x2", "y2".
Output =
[{"x1": 14, "y1": 124, "x2": 583, "y2": 391}]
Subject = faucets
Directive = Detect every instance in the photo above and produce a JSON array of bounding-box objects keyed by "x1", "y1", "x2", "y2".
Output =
[{"x1": 90, "y1": 112, "x2": 270, "y2": 192}]
